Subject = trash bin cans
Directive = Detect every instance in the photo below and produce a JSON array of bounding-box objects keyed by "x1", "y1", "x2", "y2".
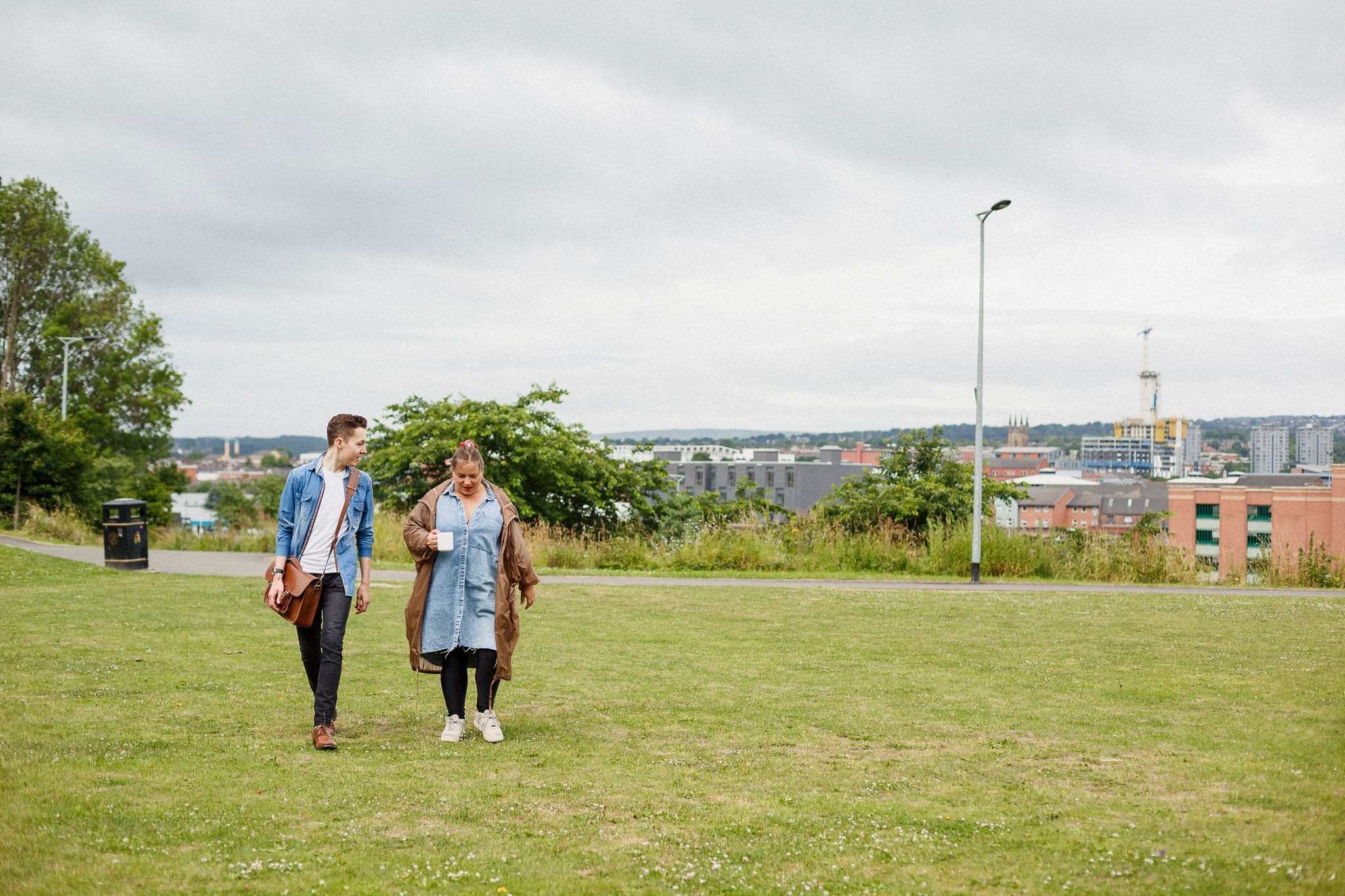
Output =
[{"x1": 102, "y1": 498, "x2": 149, "y2": 569}]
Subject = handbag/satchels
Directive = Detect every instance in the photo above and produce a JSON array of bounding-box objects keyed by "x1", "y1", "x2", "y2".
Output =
[{"x1": 263, "y1": 557, "x2": 321, "y2": 628}]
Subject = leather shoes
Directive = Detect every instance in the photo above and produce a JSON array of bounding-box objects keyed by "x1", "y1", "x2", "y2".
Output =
[
  {"x1": 329, "y1": 708, "x2": 337, "y2": 735},
  {"x1": 312, "y1": 711, "x2": 338, "y2": 749}
]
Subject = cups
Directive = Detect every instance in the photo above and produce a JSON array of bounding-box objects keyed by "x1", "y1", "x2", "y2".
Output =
[{"x1": 437, "y1": 531, "x2": 454, "y2": 552}]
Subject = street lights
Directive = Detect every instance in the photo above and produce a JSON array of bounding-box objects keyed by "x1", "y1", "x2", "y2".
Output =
[
  {"x1": 969, "y1": 198, "x2": 1011, "y2": 585},
  {"x1": 56, "y1": 336, "x2": 100, "y2": 420}
]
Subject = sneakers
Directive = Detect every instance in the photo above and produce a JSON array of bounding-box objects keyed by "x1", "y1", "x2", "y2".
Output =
[
  {"x1": 474, "y1": 709, "x2": 504, "y2": 742},
  {"x1": 441, "y1": 713, "x2": 467, "y2": 742}
]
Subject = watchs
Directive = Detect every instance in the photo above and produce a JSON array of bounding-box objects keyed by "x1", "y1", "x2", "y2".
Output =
[{"x1": 272, "y1": 568, "x2": 284, "y2": 576}]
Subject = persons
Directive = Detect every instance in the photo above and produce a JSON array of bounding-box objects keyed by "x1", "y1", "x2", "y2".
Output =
[
  {"x1": 403, "y1": 441, "x2": 541, "y2": 742},
  {"x1": 268, "y1": 414, "x2": 374, "y2": 748}
]
